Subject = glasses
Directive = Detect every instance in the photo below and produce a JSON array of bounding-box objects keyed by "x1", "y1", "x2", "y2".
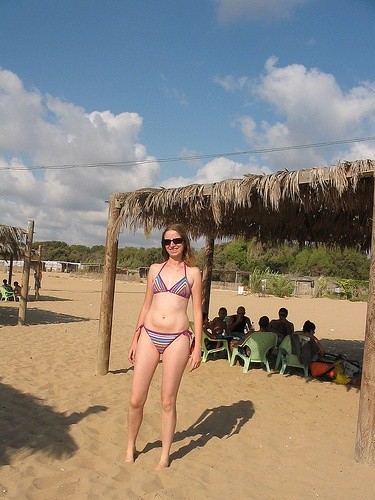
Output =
[{"x1": 163, "y1": 238, "x2": 184, "y2": 245}]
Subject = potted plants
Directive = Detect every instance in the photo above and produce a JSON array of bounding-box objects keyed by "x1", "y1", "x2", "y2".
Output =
[
  {"x1": 250, "y1": 266, "x2": 262, "y2": 297},
  {"x1": 238, "y1": 277, "x2": 245, "y2": 295}
]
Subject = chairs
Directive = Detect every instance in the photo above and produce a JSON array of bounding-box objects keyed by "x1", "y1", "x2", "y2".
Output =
[
  {"x1": 0, "y1": 286, "x2": 20, "y2": 302},
  {"x1": 189, "y1": 321, "x2": 320, "y2": 380}
]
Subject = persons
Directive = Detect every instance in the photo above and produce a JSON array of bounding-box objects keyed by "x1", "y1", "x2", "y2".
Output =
[
  {"x1": 202, "y1": 306, "x2": 325, "y2": 370},
  {"x1": 10, "y1": 281, "x2": 21, "y2": 300},
  {"x1": 34, "y1": 271, "x2": 41, "y2": 289},
  {"x1": 124, "y1": 222, "x2": 204, "y2": 471},
  {"x1": 2, "y1": 279, "x2": 12, "y2": 300}
]
